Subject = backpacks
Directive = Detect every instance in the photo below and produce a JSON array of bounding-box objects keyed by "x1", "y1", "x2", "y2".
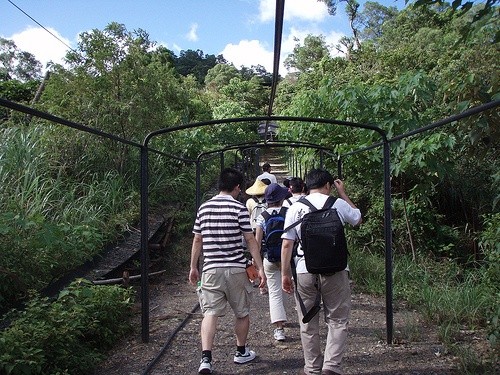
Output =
[
  {"x1": 296, "y1": 196, "x2": 349, "y2": 275},
  {"x1": 261, "y1": 207, "x2": 298, "y2": 264},
  {"x1": 249, "y1": 197, "x2": 268, "y2": 233}
]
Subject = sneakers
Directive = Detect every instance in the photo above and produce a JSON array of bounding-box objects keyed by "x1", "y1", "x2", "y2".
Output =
[
  {"x1": 233, "y1": 347, "x2": 256, "y2": 363},
  {"x1": 197, "y1": 357, "x2": 212, "y2": 373},
  {"x1": 273, "y1": 327, "x2": 286, "y2": 341}
]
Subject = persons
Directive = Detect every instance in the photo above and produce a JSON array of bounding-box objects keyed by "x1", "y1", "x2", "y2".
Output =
[
  {"x1": 282, "y1": 176, "x2": 309, "y2": 208},
  {"x1": 280, "y1": 167, "x2": 363, "y2": 375},
  {"x1": 244, "y1": 176, "x2": 269, "y2": 295},
  {"x1": 188, "y1": 167, "x2": 268, "y2": 374},
  {"x1": 282, "y1": 179, "x2": 291, "y2": 189},
  {"x1": 301, "y1": 183, "x2": 309, "y2": 195},
  {"x1": 261, "y1": 178, "x2": 272, "y2": 185},
  {"x1": 255, "y1": 182, "x2": 290, "y2": 340},
  {"x1": 256, "y1": 163, "x2": 277, "y2": 184}
]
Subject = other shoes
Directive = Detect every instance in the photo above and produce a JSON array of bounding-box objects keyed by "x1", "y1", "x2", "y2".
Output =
[{"x1": 259, "y1": 287, "x2": 268, "y2": 295}]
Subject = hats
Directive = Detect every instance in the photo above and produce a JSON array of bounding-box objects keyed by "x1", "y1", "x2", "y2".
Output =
[
  {"x1": 245, "y1": 178, "x2": 269, "y2": 195},
  {"x1": 264, "y1": 182, "x2": 288, "y2": 205}
]
